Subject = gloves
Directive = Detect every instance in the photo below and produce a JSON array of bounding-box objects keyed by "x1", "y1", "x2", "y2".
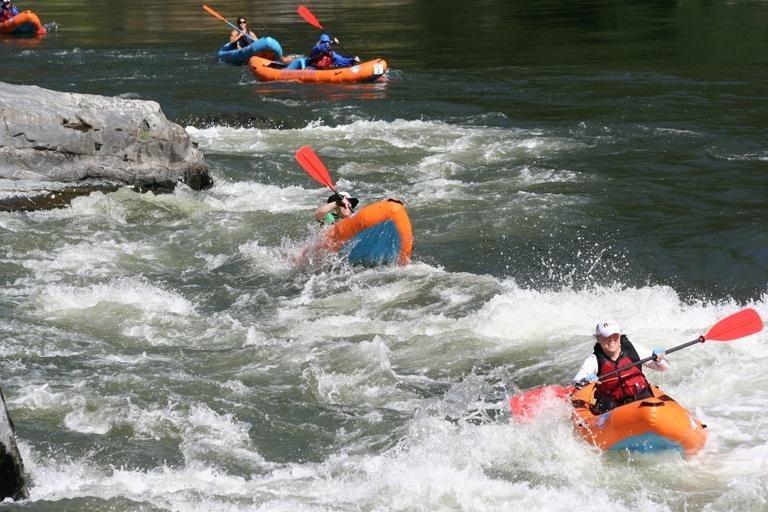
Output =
[
  {"x1": 580, "y1": 373, "x2": 603, "y2": 385},
  {"x1": 335, "y1": 194, "x2": 349, "y2": 208},
  {"x1": 352, "y1": 56, "x2": 361, "y2": 66},
  {"x1": 651, "y1": 347, "x2": 666, "y2": 362},
  {"x1": 329, "y1": 38, "x2": 339, "y2": 46}
]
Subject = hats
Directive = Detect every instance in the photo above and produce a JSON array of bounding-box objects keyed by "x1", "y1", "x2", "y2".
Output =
[
  {"x1": 595, "y1": 318, "x2": 622, "y2": 338},
  {"x1": 319, "y1": 34, "x2": 331, "y2": 42},
  {"x1": 326, "y1": 191, "x2": 360, "y2": 209}
]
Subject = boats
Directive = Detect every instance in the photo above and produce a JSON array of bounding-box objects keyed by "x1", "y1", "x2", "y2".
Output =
[
  {"x1": 298, "y1": 197, "x2": 414, "y2": 271},
  {"x1": 0, "y1": 34, "x2": 46, "y2": 48},
  {"x1": 217, "y1": 35, "x2": 283, "y2": 65},
  {"x1": 0, "y1": 10, "x2": 47, "y2": 34},
  {"x1": 510, "y1": 383, "x2": 707, "y2": 461},
  {"x1": 250, "y1": 56, "x2": 387, "y2": 84}
]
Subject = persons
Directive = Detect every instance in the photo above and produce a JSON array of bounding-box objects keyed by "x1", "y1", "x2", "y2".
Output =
[
  {"x1": 571, "y1": 320, "x2": 670, "y2": 413},
  {"x1": 316, "y1": 191, "x2": 360, "y2": 230},
  {"x1": 305, "y1": 30, "x2": 361, "y2": 70},
  {"x1": 0, "y1": 0, "x2": 18, "y2": 21},
  {"x1": 230, "y1": 16, "x2": 258, "y2": 50}
]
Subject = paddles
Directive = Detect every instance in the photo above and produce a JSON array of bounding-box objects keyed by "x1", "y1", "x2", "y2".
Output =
[
  {"x1": 511, "y1": 308, "x2": 764, "y2": 416},
  {"x1": 297, "y1": 5, "x2": 364, "y2": 65},
  {"x1": 295, "y1": 144, "x2": 354, "y2": 215},
  {"x1": 201, "y1": 3, "x2": 258, "y2": 42}
]
